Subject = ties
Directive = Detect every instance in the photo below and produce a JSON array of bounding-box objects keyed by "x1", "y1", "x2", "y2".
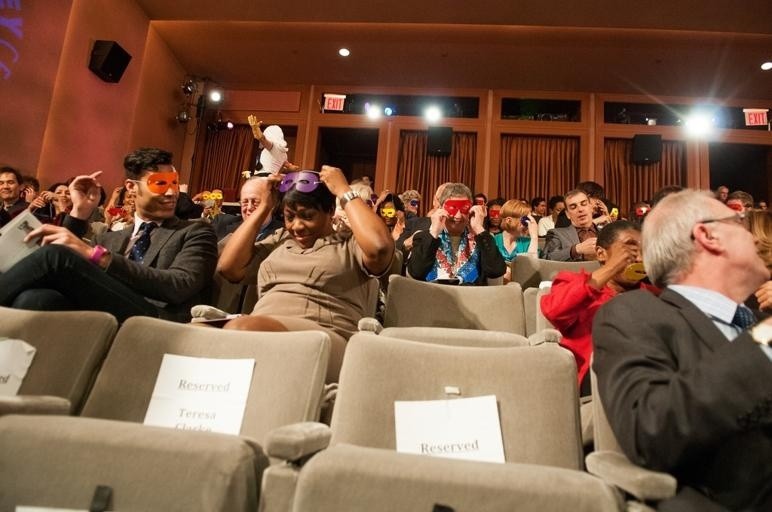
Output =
[{"x1": 128, "y1": 223, "x2": 159, "y2": 262}]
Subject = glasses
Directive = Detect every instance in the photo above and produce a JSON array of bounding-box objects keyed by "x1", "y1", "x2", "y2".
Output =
[{"x1": 691, "y1": 211, "x2": 748, "y2": 241}]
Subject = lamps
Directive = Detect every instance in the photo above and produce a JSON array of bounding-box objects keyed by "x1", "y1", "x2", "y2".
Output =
[{"x1": 177, "y1": 74, "x2": 235, "y2": 135}]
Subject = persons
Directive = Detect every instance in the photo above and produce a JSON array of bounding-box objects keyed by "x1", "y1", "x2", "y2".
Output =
[
  {"x1": 734, "y1": 210, "x2": 772, "y2": 346},
  {"x1": 590, "y1": 185, "x2": 772, "y2": 512},
  {"x1": 537, "y1": 219, "x2": 666, "y2": 398},
  {"x1": 0, "y1": 146, "x2": 217, "y2": 323},
  {"x1": 247, "y1": 115, "x2": 298, "y2": 177},
  {"x1": 1, "y1": 147, "x2": 772, "y2": 287},
  {"x1": 215, "y1": 164, "x2": 397, "y2": 382}
]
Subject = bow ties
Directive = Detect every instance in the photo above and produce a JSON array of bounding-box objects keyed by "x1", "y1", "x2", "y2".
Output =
[{"x1": 732, "y1": 306, "x2": 757, "y2": 330}]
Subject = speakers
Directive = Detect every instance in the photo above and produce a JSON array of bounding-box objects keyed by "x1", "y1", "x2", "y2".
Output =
[
  {"x1": 89, "y1": 39, "x2": 132, "y2": 83},
  {"x1": 631, "y1": 134, "x2": 661, "y2": 162},
  {"x1": 428, "y1": 127, "x2": 452, "y2": 156}
]
